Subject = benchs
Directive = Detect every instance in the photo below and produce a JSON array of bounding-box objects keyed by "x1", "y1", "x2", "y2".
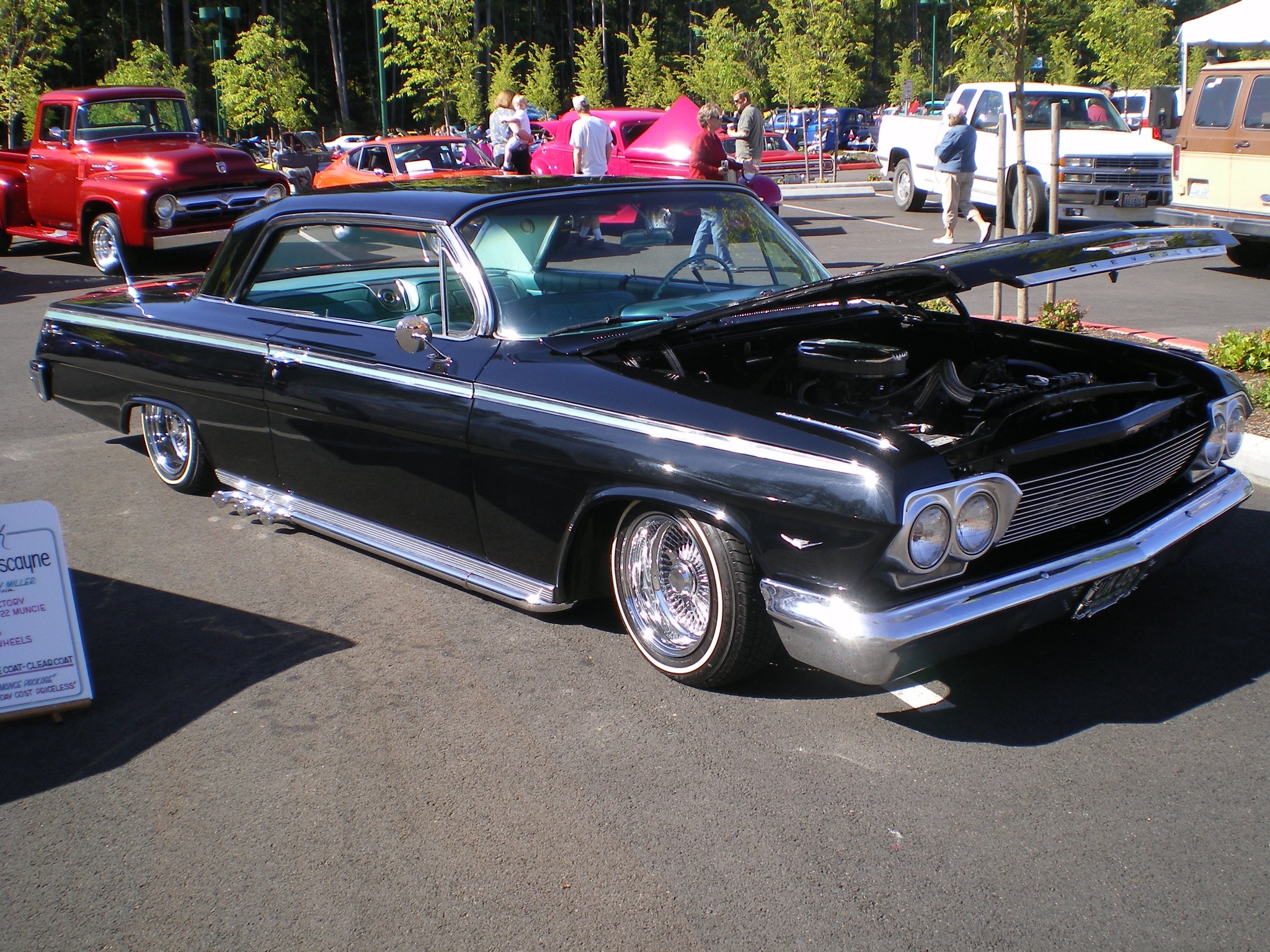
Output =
[
  {"x1": 258, "y1": 274, "x2": 521, "y2": 323},
  {"x1": 64, "y1": 124, "x2": 154, "y2": 140}
]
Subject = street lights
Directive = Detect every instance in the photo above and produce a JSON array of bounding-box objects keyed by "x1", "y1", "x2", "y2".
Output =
[
  {"x1": 198, "y1": 5, "x2": 243, "y2": 147},
  {"x1": 211, "y1": 37, "x2": 227, "y2": 138},
  {"x1": 919, "y1": 0, "x2": 951, "y2": 115}
]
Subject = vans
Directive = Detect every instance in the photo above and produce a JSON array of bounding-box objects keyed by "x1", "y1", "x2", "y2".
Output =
[
  {"x1": 1106, "y1": 90, "x2": 1191, "y2": 143},
  {"x1": 1153, "y1": 59, "x2": 1268, "y2": 264},
  {"x1": 312, "y1": 133, "x2": 521, "y2": 257}
]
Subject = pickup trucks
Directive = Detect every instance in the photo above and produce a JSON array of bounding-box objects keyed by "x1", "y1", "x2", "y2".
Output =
[
  {"x1": 873, "y1": 80, "x2": 1178, "y2": 235},
  {"x1": 0, "y1": 85, "x2": 294, "y2": 277}
]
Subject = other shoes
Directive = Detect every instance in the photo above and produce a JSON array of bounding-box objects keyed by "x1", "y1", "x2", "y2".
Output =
[
  {"x1": 573, "y1": 234, "x2": 584, "y2": 243},
  {"x1": 501, "y1": 165, "x2": 512, "y2": 170},
  {"x1": 932, "y1": 237, "x2": 954, "y2": 244},
  {"x1": 585, "y1": 238, "x2": 605, "y2": 250},
  {"x1": 980, "y1": 222, "x2": 992, "y2": 243}
]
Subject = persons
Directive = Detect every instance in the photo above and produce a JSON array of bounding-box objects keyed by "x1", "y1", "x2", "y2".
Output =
[
  {"x1": 877, "y1": 101, "x2": 928, "y2": 125},
  {"x1": 932, "y1": 103, "x2": 992, "y2": 243},
  {"x1": 688, "y1": 102, "x2": 759, "y2": 271},
  {"x1": 500, "y1": 95, "x2": 531, "y2": 171},
  {"x1": 839, "y1": 132, "x2": 861, "y2": 149},
  {"x1": 438, "y1": 125, "x2": 457, "y2": 152},
  {"x1": 807, "y1": 124, "x2": 832, "y2": 154},
  {"x1": 334, "y1": 145, "x2": 344, "y2": 154},
  {"x1": 569, "y1": 95, "x2": 613, "y2": 175},
  {"x1": 727, "y1": 89, "x2": 763, "y2": 175},
  {"x1": 533, "y1": 115, "x2": 556, "y2": 141},
  {"x1": 1087, "y1": 82, "x2": 1121, "y2": 122},
  {"x1": 375, "y1": 127, "x2": 382, "y2": 140},
  {"x1": 474, "y1": 126, "x2": 483, "y2": 136},
  {"x1": 489, "y1": 90, "x2": 533, "y2": 171}
]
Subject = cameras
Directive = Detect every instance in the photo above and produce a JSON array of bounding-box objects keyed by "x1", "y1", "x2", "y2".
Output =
[{"x1": 721, "y1": 160, "x2": 729, "y2": 169}]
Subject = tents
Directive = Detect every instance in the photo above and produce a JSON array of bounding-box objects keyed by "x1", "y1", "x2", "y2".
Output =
[{"x1": 1174, "y1": 0, "x2": 1270, "y2": 114}]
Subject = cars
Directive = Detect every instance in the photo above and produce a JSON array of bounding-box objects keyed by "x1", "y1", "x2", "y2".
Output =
[
  {"x1": 25, "y1": 172, "x2": 1257, "y2": 693},
  {"x1": 719, "y1": 97, "x2": 950, "y2": 155},
  {"x1": 460, "y1": 94, "x2": 787, "y2": 236},
  {"x1": 322, "y1": 134, "x2": 371, "y2": 155}
]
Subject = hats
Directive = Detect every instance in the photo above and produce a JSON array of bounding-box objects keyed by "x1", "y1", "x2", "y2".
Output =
[
  {"x1": 572, "y1": 95, "x2": 589, "y2": 109},
  {"x1": 1099, "y1": 81, "x2": 1117, "y2": 92},
  {"x1": 940, "y1": 103, "x2": 966, "y2": 116}
]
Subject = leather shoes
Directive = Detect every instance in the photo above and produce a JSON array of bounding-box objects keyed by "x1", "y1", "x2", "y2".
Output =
[
  {"x1": 692, "y1": 263, "x2": 715, "y2": 270},
  {"x1": 723, "y1": 264, "x2": 740, "y2": 272}
]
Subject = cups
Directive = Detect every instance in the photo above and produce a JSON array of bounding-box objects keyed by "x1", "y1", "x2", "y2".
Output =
[{"x1": 726, "y1": 123, "x2": 735, "y2": 130}]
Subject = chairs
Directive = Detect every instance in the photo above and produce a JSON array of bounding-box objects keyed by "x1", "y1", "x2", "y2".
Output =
[
  {"x1": 501, "y1": 292, "x2": 638, "y2": 336},
  {"x1": 371, "y1": 306, "x2": 475, "y2": 335}
]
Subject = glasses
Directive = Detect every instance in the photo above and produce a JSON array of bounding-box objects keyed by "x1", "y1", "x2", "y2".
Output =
[
  {"x1": 734, "y1": 96, "x2": 744, "y2": 103},
  {"x1": 712, "y1": 115, "x2": 722, "y2": 119}
]
